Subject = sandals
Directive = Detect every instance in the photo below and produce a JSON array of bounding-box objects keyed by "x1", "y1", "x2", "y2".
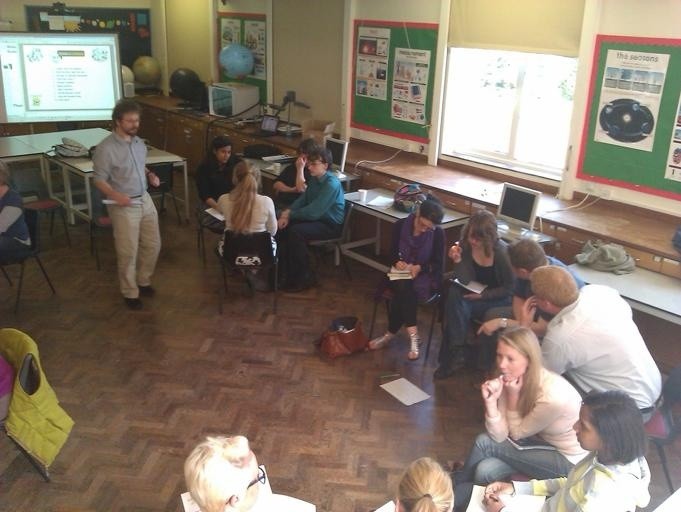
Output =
[
  {"x1": 408, "y1": 334, "x2": 423, "y2": 361},
  {"x1": 369, "y1": 332, "x2": 394, "y2": 350}
]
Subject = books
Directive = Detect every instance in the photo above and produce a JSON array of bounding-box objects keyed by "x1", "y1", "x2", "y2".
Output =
[
  {"x1": 453, "y1": 276, "x2": 488, "y2": 295},
  {"x1": 387, "y1": 272, "x2": 410, "y2": 277},
  {"x1": 391, "y1": 263, "x2": 414, "y2": 274},
  {"x1": 465, "y1": 481, "x2": 546, "y2": 512},
  {"x1": 101, "y1": 198, "x2": 144, "y2": 206},
  {"x1": 366, "y1": 196, "x2": 395, "y2": 210},
  {"x1": 205, "y1": 206, "x2": 226, "y2": 221},
  {"x1": 507, "y1": 434, "x2": 556, "y2": 451},
  {"x1": 180, "y1": 463, "x2": 316, "y2": 512},
  {"x1": 389, "y1": 275, "x2": 412, "y2": 281}
]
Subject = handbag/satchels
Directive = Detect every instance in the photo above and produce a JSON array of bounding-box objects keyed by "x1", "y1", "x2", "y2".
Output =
[
  {"x1": 319, "y1": 317, "x2": 367, "y2": 357},
  {"x1": 395, "y1": 184, "x2": 426, "y2": 213}
]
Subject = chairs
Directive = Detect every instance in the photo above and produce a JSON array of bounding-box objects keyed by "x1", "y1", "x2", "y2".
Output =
[
  {"x1": 643, "y1": 367, "x2": 681, "y2": 495},
  {"x1": 0, "y1": 326, "x2": 49, "y2": 483}
]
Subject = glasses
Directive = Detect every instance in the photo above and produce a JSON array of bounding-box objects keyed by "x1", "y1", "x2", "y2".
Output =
[{"x1": 247, "y1": 464, "x2": 266, "y2": 488}]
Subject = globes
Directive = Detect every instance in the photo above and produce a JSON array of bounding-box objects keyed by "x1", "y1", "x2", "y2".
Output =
[
  {"x1": 120, "y1": 64, "x2": 135, "y2": 84},
  {"x1": 133, "y1": 56, "x2": 162, "y2": 95},
  {"x1": 218, "y1": 44, "x2": 255, "y2": 79},
  {"x1": 169, "y1": 67, "x2": 201, "y2": 109}
]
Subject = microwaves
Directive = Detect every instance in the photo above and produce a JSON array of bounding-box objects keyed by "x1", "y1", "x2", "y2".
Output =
[{"x1": 209, "y1": 83, "x2": 260, "y2": 119}]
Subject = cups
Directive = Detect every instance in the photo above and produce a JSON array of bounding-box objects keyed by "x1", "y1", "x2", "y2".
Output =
[
  {"x1": 359, "y1": 189, "x2": 368, "y2": 203},
  {"x1": 274, "y1": 163, "x2": 281, "y2": 174}
]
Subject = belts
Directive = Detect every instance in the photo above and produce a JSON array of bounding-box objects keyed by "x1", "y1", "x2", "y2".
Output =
[{"x1": 641, "y1": 394, "x2": 661, "y2": 415}]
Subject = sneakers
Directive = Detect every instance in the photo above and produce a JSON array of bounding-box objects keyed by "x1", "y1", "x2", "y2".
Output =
[
  {"x1": 433, "y1": 363, "x2": 455, "y2": 379},
  {"x1": 126, "y1": 298, "x2": 142, "y2": 310},
  {"x1": 138, "y1": 285, "x2": 154, "y2": 297}
]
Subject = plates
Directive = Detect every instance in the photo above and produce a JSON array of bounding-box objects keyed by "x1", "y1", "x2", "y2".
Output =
[{"x1": 278, "y1": 125, "x2": 303, "y2": 132}]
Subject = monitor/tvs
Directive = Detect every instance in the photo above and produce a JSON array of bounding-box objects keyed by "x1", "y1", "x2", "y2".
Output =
[
  {"x1": 495, "y1": 179, "x2": 543, "y2": 238},
  {"x1": 323, "y1": 135, "x2": 349, "y2": 180}
]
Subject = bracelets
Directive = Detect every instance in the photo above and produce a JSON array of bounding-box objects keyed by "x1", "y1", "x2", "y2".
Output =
[
  {"x1": 501, "y1": 317, "x2": 507, "y2": 328},
  {"x1": 145, "y1": 170, "x2": 155, "y2": 177}
]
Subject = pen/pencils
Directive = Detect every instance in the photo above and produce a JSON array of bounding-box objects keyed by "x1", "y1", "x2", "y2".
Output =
[{"x1": 129, "y1": 195, "x2": 142, "y2": 199}]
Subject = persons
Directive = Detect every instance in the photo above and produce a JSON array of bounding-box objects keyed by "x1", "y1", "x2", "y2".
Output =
[
  {"x1": 278, "y1": 146, "x2": 346, "y2": 292},
  {"x1": 92, "y1": 99, "x2": 164, "y2": 310},
  {"x1": 271, "y1": 138, "x2": 320, "y2": 218},
  {"x1": 396, "y1": 456, "x2": 453, "y2": 512},
  {"x1": 367, "y1": 191, "x2": 446, "y2": 360},
  {"x1": 0, "y1": 161, "x2": 34, "y2": 266},
  {"x1": 215, "y1": 158, "x2": 279, "y2": 277},
  {"x1": 1, "y1": 356, "x2": 16, "y2": 425},
  {"x1": 185, "y1": 434, "x2": 266, "y2": 512},
  {"x1": 433, "y1": 210, "x2": 518, "y2": 381},
  {"x1": 194, "y1": 136, "x2": 248, "y2": 233},
  {"x1": 451, "y1": 325, "x2": 600, "y2": 512},
  {"x1": 455, "y1": 390, "x2": 652, "y2": 512},
  {"x1": 473, "y1": 238, "x2": 587, "y2": 376},
  {"x1": 521, "y1": 264, "x2": 666, "y2": 425}
]
